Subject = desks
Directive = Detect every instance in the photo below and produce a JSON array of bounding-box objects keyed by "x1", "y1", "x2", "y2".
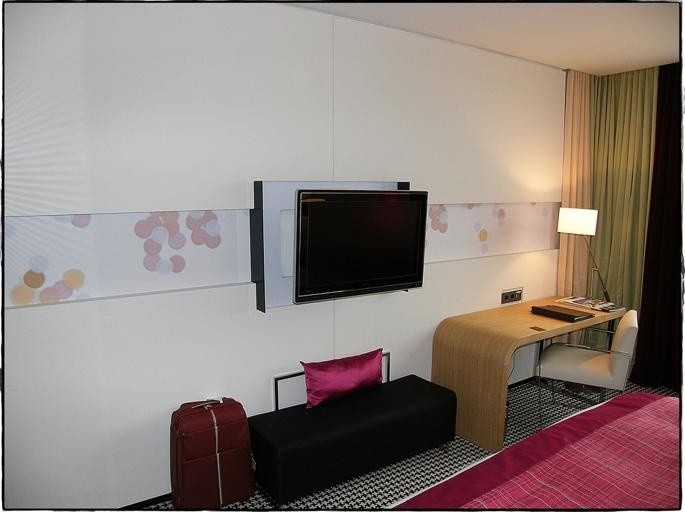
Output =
[{"x1": 432, "y1": 295, "x2": 626, "y2": 453}]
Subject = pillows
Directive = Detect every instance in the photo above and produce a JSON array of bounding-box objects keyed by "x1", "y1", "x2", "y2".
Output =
[{"x1": 300, "y1": 348, "x2": 383, "y2": 409}]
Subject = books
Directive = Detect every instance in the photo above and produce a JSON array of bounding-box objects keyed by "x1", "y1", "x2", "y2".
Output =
[
  {"x1": 531, "y1": 305, "x2": 595, "y2": 323},
  {"x1": 554, "y1": 294, "x2": 627, "y2": 313}
]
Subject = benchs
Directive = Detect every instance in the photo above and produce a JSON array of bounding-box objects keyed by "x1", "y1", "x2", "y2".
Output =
[{"x1": 247, "y1": 373, "x2": 457, "y2": 507}]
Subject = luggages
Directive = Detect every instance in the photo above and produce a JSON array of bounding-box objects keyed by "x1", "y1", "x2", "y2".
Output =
[{"x1": 170, "y1": 399, "x2": 256, "y2": 510}]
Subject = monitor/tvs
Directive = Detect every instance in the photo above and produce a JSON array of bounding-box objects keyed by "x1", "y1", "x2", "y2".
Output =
[{"x1": 293, "y1": 189, "x2": 428, "y2": 304}]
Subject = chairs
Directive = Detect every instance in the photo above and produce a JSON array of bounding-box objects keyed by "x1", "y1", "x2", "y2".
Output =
[{"x1": 535, "y1": 309, "x2": 639, "y2": 430}]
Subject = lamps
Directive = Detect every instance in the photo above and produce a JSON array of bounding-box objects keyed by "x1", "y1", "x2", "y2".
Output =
[{"x1": 557, "y1": 206, "x2": 610, "y2": 302}]
